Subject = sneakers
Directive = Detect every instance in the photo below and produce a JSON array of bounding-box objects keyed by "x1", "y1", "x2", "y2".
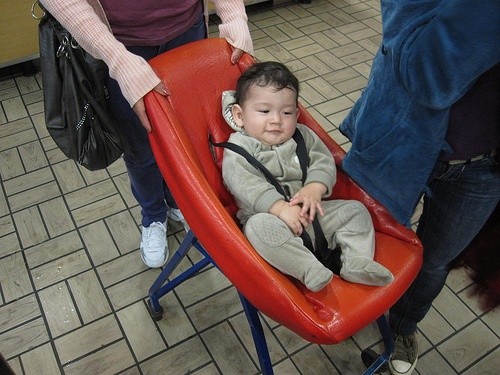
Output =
[
  {"x1": 167, "y1": 207, "x2": 190, "y2": 232},
  {"x1": 140, "y1": 217, "x2": 169, "y2": 268}
]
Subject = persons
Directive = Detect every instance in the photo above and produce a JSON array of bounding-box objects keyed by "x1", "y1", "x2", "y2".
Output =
[
  {"x1": 340, "y1": 0, "x2": 500, "y2": 375},
  {"x1": 222, "y1": 61, "x2": 395, "y2": 293},
  {"x1": 39, "y1": 0, "x2": 254, "y2": 268}
]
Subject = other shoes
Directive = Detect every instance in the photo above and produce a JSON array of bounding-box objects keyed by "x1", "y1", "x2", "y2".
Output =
[{"x1": 389, "y1": 331, "x2": 418, "y2": 375}]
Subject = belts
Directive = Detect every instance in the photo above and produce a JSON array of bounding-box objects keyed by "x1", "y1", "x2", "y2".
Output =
[{"x1": 449, "y1": 151, "x2": 496, "y2": 165}]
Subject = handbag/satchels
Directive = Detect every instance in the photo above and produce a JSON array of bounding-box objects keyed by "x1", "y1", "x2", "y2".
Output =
[{"x1": 38, "y1": 16, "x2": 123, "y2": 172}]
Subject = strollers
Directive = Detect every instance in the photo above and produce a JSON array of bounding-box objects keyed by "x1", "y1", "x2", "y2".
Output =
[{"x1": 143, "y1": 36, "x2": 424, "y2": 375}]
